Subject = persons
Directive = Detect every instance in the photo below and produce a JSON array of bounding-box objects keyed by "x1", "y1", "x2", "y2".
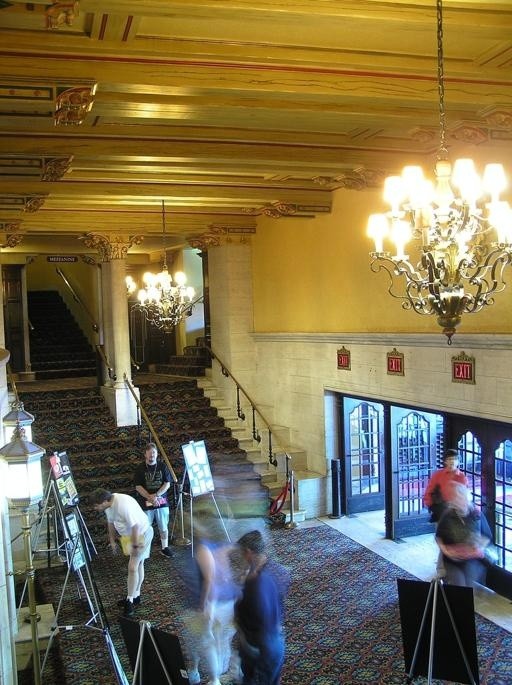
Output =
[
  {"x1": 133, "y1": 442, "x2": 175, "y2": 559},
  {"x1": 88, "y1": 487, "x2": 155, "y2": 616},
  {"x1": 230, "y1": 531, "x2": 288, "y2": 685},
  {"x1": 175, "y1": 512, "x2": 226, "y2": 684},
  {"x1": 434, "y1": 482, "x2": 493, "y2": 592},
  {"x1": 424, "y1": 448, "x2": 469, "y2": 574}
]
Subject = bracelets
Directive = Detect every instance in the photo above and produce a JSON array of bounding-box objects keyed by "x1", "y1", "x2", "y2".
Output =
[
  {"x1": 131, "y1": 545, "x2": 138, "y2": 549},
  {"x1": 156, "y1": 492, "x2": 159, "y2": 497}
]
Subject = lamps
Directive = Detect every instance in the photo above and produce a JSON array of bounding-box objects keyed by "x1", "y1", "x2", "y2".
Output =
[
  {"x1": 2, "y1": 378, "x2": 48, "y2": 685},
  {"x1": 137, "y1": 199, "x2": 196, "y2": 335},
  {"x1": 366, "y1": 0, "x2": 511, "y2": 347}
]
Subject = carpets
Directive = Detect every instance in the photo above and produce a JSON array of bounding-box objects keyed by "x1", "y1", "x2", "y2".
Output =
[
  {"x1": 15, "y1": 381, "x2": 512, "y2": 684},
  {"x1": 29, "y1": 288, "x2": 214, "y2": 380}
]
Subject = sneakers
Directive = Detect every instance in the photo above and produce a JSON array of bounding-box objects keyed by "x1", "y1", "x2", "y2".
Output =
[
  {"x1": 117, "y1": 595, "x2": 140, "y2": 616},
  {"x1": 161, "y1": 547, "x2": 174, "y2": 558},
  {"x1": 188, "y1": 671, "x2": 221, "y2": 685}
]
